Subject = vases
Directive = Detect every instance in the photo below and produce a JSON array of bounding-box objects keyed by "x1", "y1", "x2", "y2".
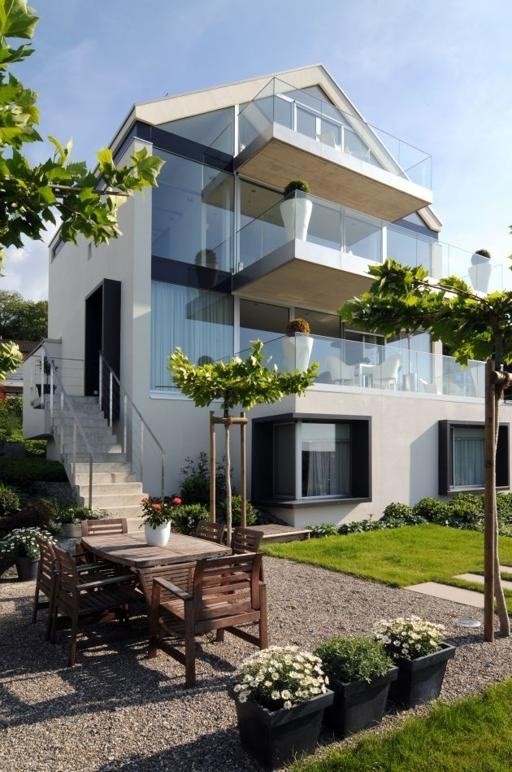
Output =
[
  {"x1": 13, "y1": 554, "x2": 41, "y2": 581},
  {"x1": 392, "y1": 641, "x2": 457, "y2": 705},
  {"x1": 233, "y1": 687, "x2": 336, "y2": 771}
]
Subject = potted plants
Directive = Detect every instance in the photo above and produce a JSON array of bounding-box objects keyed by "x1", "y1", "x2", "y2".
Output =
[
  {"x1": 467, "y1": 248, "x2": 493, "y2": 290},
  {"x1": 283, "y1": 320, "x2": 317, "y2": 376},
  {"x1": 279, "y1": 178, "x2": 313, "y2": 241},
  {"x1": 311, "y1": 634, "x2": 399, "y2": 739},
  {"x1": 55, "y1": 504, "x2": 94, "y2": 536}
]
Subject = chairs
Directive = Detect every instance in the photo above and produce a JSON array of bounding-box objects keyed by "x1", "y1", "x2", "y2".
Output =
[
  {"x1": 33, "y1": 519, "x2": 268, "y2": 689},
  {"x1": 328, "y1": 353, "x2": 404, "y2": 389}
]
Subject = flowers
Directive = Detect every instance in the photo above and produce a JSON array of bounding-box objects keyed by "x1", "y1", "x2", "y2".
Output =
[
  {"x1": 2, "y1": 528, "x2": 55, "y2": 558},
  {"x1": 230, "y1": 647, "x2": 326, "y2": 709},
  {"x1": 138, "y1": 495, "x2": 181, "y2": 525},
  {"x1": 370, "y1": 612, "x2": 444, "y2": 661}
]
